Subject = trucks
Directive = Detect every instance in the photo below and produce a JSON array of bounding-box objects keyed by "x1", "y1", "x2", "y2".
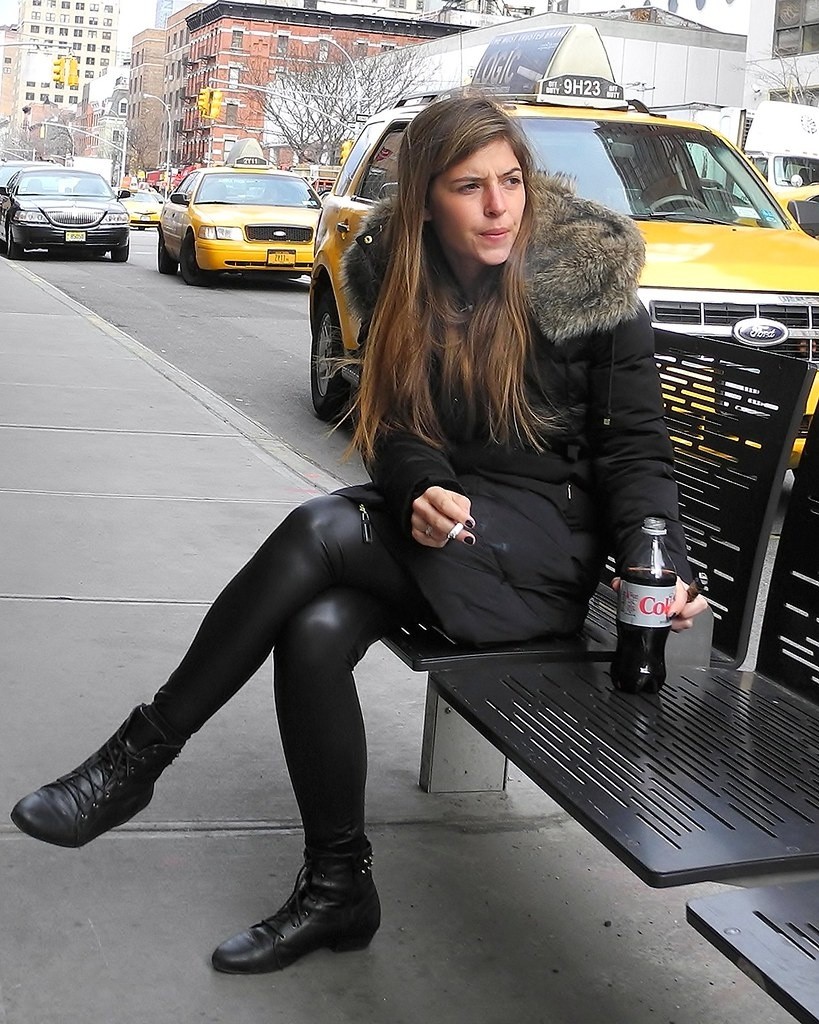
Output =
[{"x1": 64, "y1": 155, "x2": 114, "y2": 187}]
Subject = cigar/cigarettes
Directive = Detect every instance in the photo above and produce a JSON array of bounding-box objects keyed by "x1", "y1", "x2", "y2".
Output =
[{"x1": 447, "y1": 521, "x2": 465, "y2": 539}]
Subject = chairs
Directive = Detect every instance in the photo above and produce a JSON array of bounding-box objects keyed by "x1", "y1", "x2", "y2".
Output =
[
  {"x1": 202, "y1": 181, "x2": 227, "y2": 202},
  {"x1": 26, "y1": 179, "x2": 46, "y2": 194}
]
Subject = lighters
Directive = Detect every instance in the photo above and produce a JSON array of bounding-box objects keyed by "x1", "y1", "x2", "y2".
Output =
[{"x1": 685, "y1": 572, "x2": 707, "y2": 602}]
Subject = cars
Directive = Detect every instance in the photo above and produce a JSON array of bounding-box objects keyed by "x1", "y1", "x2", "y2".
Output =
[
  {"x1": 309, "y1": 24, "x2": 819, "y2": 477},
  {"x1": 158, "y1": 138, "x2": 322, "y2": 288},
  {"x1": 0, "y1": 166, "x2": 132, "y2": 263},
  {"x1": 109, "y1": 177, "x2": 167, "y2": 231}
]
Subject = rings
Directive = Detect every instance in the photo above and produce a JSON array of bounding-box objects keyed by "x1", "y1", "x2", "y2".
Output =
[{"x1": 425, "y1": 525, "x2": 434, "y2": 537}]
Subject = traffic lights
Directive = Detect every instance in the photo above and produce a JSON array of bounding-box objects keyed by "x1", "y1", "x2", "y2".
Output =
[
  {"x1": 197, "y1": 87, "x2": 213, "y2": 112},
  {"x1": 339, "y1": 141, "x2": 351, "y2": 167},
  {"x1": 52, "y1": 57, "x2": 66, "y2": 84}
]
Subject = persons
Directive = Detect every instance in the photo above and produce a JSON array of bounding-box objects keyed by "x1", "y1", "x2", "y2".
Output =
[{"x1": 10, "y1": 104, "x2": 710, "y2": 980}]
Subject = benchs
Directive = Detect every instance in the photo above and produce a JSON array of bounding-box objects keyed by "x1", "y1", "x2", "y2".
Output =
[{"x1": 380, "y1": 326, "x2": 819, "y2": 1024}]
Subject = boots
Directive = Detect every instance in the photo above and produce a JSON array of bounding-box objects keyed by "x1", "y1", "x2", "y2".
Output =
[
  {"x1": 211, "y1": 838, "x2": 381, "y2": 978},
  {"x1": 9, "y1": 705, "x2": 187, "y2": 849}
]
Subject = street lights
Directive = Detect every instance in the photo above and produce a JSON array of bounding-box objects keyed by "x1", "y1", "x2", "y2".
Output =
[
  {"x1": 299, "y1": 36, "x2": 361, "y2": 140},
  {"x1": 143, "y1": 93, "x2": 171, "y2": 193}
]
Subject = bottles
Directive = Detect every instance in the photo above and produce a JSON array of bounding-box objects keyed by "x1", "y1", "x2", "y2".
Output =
[{"x1": 610, "y1": 518, "x2": 678, "y2": 694}]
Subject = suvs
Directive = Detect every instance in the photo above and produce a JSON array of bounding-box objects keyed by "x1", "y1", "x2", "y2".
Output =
[{"x1": 0, "y1": 159, "x2": 75, "y2": 194}]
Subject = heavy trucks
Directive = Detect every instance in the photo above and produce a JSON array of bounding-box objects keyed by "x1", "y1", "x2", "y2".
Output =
[{"x1": 643, "y1": 101, "x2": 819, "y2": 222}]
